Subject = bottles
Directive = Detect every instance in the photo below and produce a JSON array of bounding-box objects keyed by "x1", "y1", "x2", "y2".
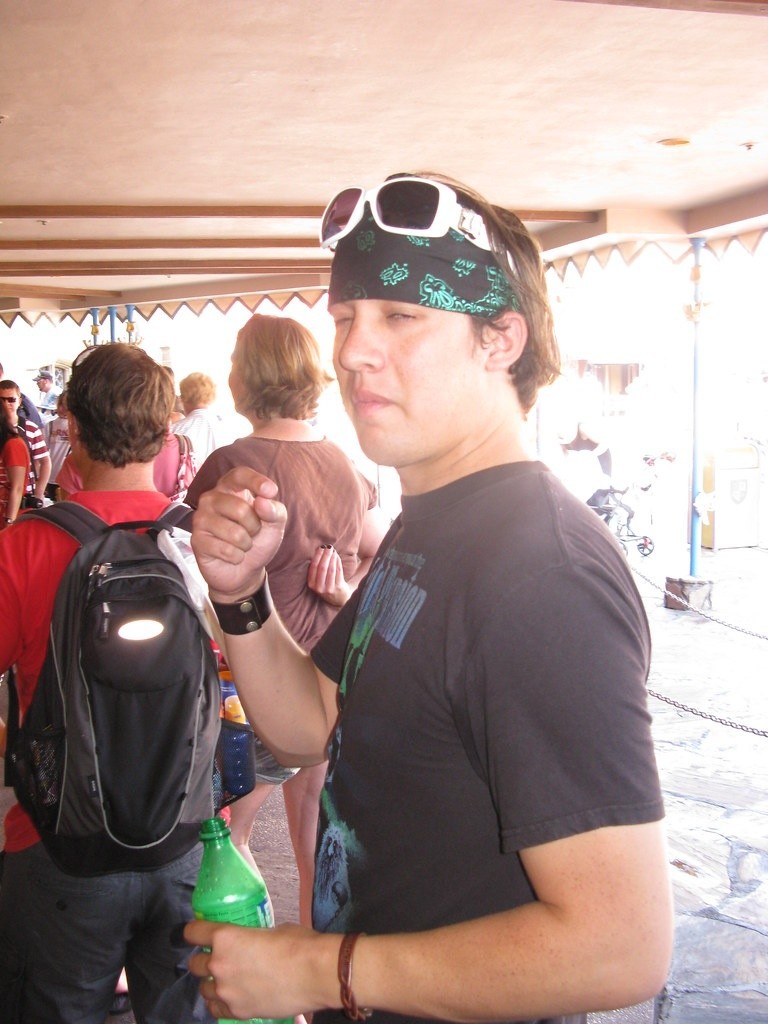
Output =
[{"x1": 191, "y1": 814, "x2": 295, "y2": 1024}]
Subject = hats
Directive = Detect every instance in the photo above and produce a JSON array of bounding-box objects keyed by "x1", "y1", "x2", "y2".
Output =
[{"x1": 32, "y1": 372, "x2": 53, "y2": 381}]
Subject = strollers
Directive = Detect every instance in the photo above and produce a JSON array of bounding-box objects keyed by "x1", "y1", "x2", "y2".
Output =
[{"x1": 569, "y1": 452, "x2": 675, "y2": 559}]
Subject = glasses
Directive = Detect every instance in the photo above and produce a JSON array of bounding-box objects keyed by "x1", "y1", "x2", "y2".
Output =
[
  {"x1": 0, "y1": 396, "x2": 19, "y2": 403},
  {"x1": 317, "y1": 176, "x2": 516, "y2": 273},
  {"x1": 72, "y1": 344, "x2": 107, "y2": 379}
]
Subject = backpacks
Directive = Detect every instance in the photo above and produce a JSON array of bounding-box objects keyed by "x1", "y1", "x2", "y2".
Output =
[{"x1": 9, "y1": 501, "x2": 255, "y2": 879}]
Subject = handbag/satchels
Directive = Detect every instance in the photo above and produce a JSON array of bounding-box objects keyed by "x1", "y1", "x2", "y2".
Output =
[{"x1": 168, "y1": 433, "x2": 198, "y2": 504}]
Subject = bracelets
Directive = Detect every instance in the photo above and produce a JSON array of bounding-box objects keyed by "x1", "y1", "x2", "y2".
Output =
[
  {"x1": 4, "y1": 517, "x2": 14, "y2": 525},
  {"x1": 205, "y1": 575, "x2": 276, "y2": 635},
  {"x1": 336, "y1": 928, "x2": 374, "y2": 1020}
]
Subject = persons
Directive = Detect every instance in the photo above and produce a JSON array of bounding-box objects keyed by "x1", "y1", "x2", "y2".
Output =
[
  {"x1": 178, "y1": 312, "x2": 385, "y2": 1024},
  {"x1": 0, "y1": 342, "x2": 220, "y2": 1024},
  {"x1": 188, "y1": 172, "x2": 674, "y2": 1024},
  {"x1": 0, "y1": 360, "x2": 217, "y2": 529}
]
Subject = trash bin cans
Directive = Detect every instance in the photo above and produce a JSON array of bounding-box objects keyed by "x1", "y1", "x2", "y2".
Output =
[{"x1": 700, "y1": 444, "x2": 762, "y2": 549}]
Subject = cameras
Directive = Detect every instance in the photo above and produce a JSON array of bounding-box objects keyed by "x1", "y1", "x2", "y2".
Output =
[{"x1": 19, "y1": 493, "x2": 43, "y2": 510}]
